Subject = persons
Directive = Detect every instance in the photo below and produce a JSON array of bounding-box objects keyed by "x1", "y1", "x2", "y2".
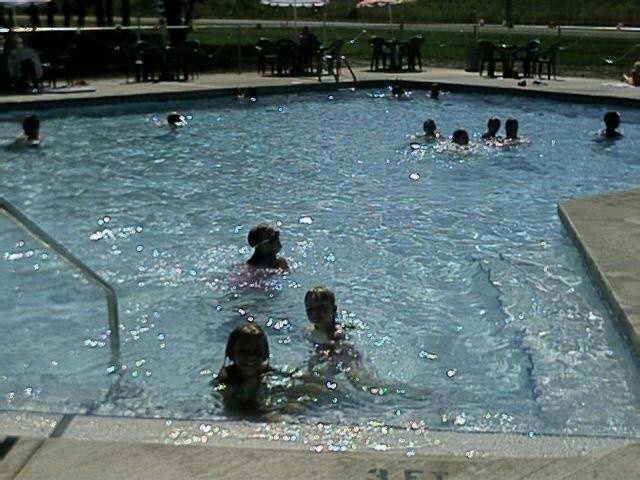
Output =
[
  {"x1": 168, "y1": 112, "x2": 181, "y2": 123},
  {"x1": 23, "y1": 116, "x2": 39, "y2": 140},
  {"x1": 602, "y1": 111, "x2": 623, "y2": 137},
  {"x1": 290, "y1": 27, "x2": 319, "y2": 74},
  {"x1": 450, "y1": 129, "x2": 469, "y2": 145},
  {"x1": 502, "y1": 118, "x2": 523, "y2": 143},
  {"x1": 421, "y1": 119, "x2": 444, "y2": 139},
  {"x1": 246, "y1": 224, "x2": 288, "y2": 270},
  {"x1": 482, "y1": 117, "x2": 502, "y2": 142},
  {"x1": 431, "y1": 83, "x2": 439, "y2": 100},
  {"x1": 301, "y1": 287, "x2": 433, "y2": 399},
  {"x1": 210, "y1": 325, "x2": 344, "y2": 423}
]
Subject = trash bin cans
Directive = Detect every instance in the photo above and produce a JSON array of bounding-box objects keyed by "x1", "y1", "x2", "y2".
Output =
[{"x1": 464, "y1": 47, "x2": 480, "y2": 72}]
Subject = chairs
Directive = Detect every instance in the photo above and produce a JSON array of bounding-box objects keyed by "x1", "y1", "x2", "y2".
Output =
[
  {"x1": 476, "y1": 38, "x2": 560, "y2": 78},
  {"x1": 255, "y1": 35, "x2": 359, "y2": 84},
  {"x1": 48, "y1": 30, "x2": 82, "y2": 88},
  {"x1": 367, "y1": 34, "x2": 425, "y2": 71},
  {"x1": 133, "y1": 40, "x2": 194, "y2": 82}
]
